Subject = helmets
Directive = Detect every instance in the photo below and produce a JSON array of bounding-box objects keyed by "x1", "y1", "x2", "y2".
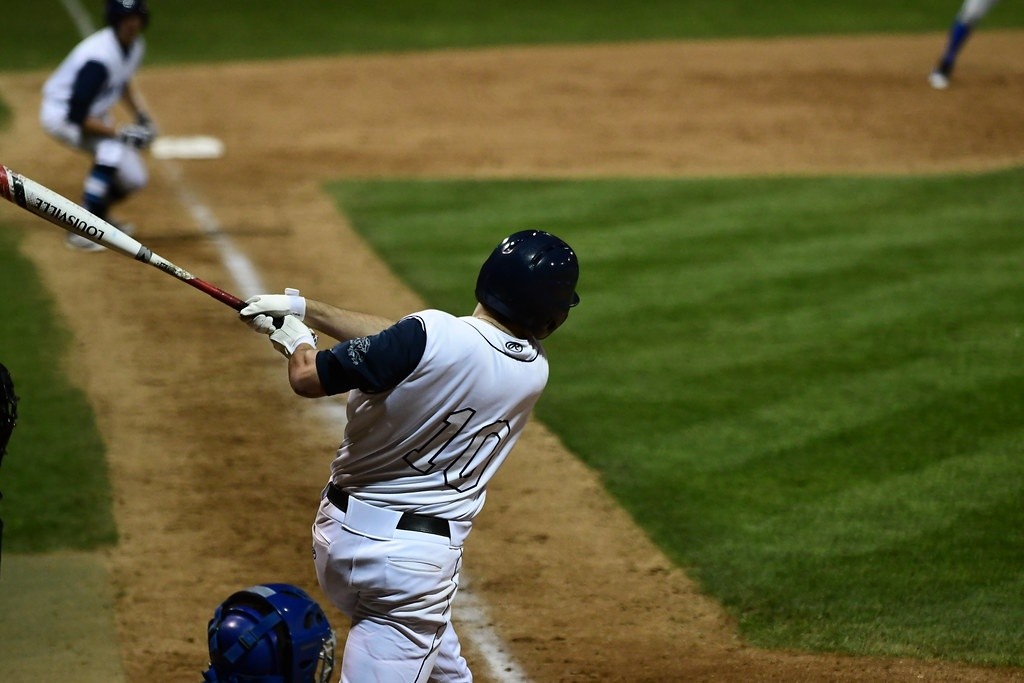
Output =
[
  {"x1": 474, "y1": 228, "x2": 580, "y2": 340},
  {"x1": 202, "y1": 582, "x2": 336, "y2": 683},
  {"x1": 106, "y1": 0, "x2": 151, "y2": 30}
]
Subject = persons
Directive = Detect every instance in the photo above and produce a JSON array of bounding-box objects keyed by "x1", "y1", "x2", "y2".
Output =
[
  {"x1": 240, "y1": 230, "x2": 580, "y2": 683},
  {"x1": 932, "y1": 1, "x2": 988, "y2": 87},
  {"x1": 39, "y1": 0, "x2": 161, "y2": 254}
]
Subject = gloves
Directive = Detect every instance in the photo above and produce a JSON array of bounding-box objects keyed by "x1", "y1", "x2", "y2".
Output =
[
  {"x1": 269, "y1": 314, "x2": 317, "y2": 359},
  {"x1": 118, "y1": 122, "x2": 149, "y2": 147},
  {"x1": 239, "y1": 287, "x2": 306, "y2": 335}
]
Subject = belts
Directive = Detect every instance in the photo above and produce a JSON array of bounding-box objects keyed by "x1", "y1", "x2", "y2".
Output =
[{"x1": 326, "y1": 483, "x2": 452, "y2": 536}]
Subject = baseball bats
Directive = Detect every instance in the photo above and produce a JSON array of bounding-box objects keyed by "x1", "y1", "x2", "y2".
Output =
[{"x1": 0, "y1": 161, "x2": 322, "y2": 351}]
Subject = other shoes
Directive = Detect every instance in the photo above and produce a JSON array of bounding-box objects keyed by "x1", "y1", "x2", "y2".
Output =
[
  {"x1": 929, "y1": 68, "x2": 948, "y2": 90},
  {"x1": 66, "y1": 233, "x2": 106, "y2": 252}
]
